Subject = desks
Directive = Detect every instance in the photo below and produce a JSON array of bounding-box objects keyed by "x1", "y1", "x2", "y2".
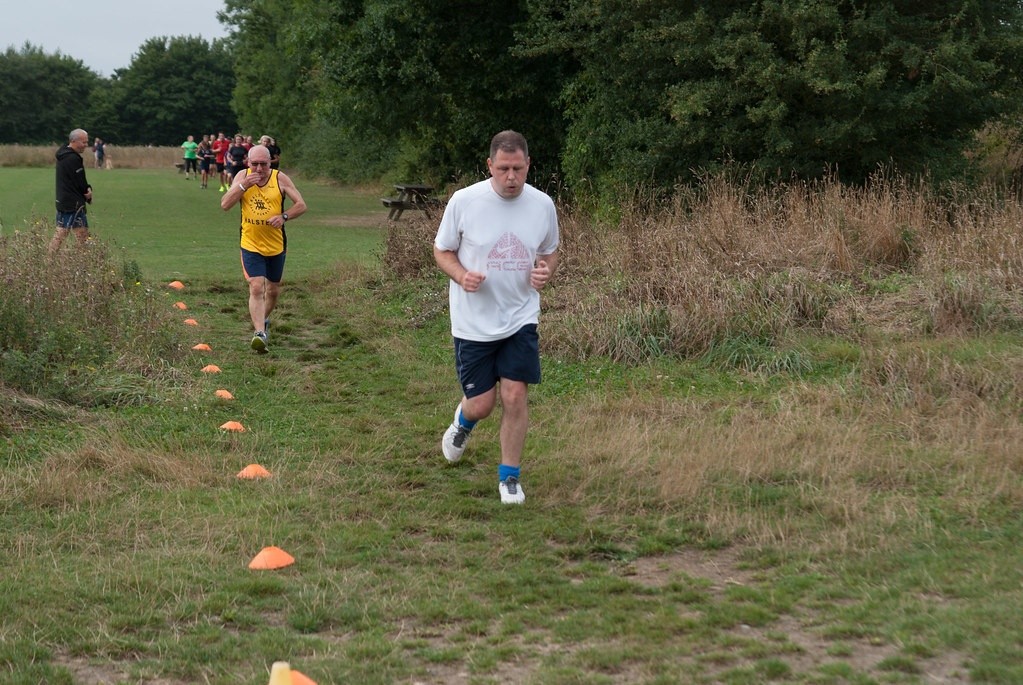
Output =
[{"x1": 384, "y1": 181, "x2": 436, "y2": 228}]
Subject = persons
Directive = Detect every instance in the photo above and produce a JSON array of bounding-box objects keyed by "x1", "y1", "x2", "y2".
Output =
[
  {"x1": 48, "y1": 129, "x2": 93, "y2": 255},
  {"x1": 219, "y1": 145, "x2": 307, "y2": 355},
  {"x1": 432, "y1": 129, "x2": 561, "y2": 504},
  {"x1": 179, "y1": 131, "x2": 281, "y2": 193},
  {"x1": 93, "y1": 137, "x2": 114, "y2": 170}
]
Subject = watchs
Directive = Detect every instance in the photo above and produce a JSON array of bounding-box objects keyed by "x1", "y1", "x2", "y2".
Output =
[{"x1": 281, "y1": 213, "x2": 288, "y2": 222}]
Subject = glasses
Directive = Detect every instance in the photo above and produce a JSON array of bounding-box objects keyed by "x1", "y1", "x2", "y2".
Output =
[{"x1": 249, "y1": 160, "x2": 271, "y2": 166}]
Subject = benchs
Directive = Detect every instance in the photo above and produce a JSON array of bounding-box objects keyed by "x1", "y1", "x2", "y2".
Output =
[{"x1": 380, "y1": 197, "x2": 402, "y2": 211}]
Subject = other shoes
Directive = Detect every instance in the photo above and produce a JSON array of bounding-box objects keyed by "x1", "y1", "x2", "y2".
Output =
[{"x1": 183, "y1": 171, "x2": 216, "y2": 190}]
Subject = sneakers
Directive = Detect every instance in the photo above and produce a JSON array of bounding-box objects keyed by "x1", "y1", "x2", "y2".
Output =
[
  {"x1": 225, "y1": 183, "x2": 231, "y2": 191},
  {"x1": 441, "y1": 401, "x2": 481, "y2": 463},
  {"x1": 218, "y1": 185, "x2": 224, "y2": 193},
  {"x1": 250, "y1": 331, "x2": 269, "y2": 354},
  {"x1": 499, "y1": 475, "x2": 526, "y2": 505}
]
